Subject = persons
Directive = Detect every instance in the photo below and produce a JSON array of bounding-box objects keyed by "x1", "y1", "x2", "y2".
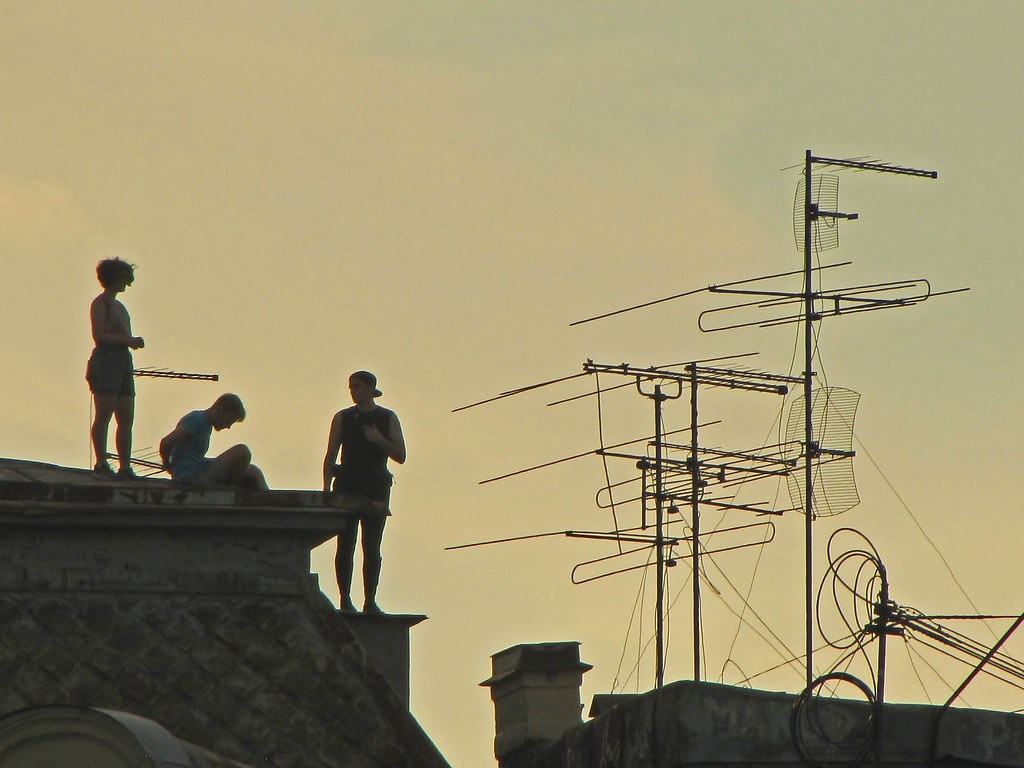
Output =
[
  {"x1": 160, "y1": 392, "x2": 269, "y2": 490},
  {"x1": 322, "y1": 371, "x2": 406, "y2": 613},
  {"x1": 85, "y1": 257, "x2": 145, "y2": 479}
]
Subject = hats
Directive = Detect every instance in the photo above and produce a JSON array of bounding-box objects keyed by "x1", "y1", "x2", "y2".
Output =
[{"x1": 349, "y1": 371, "x2": 382, "y2": 398}]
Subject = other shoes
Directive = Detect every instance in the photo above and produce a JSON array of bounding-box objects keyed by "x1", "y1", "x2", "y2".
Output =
[
  {"x1": 363, "y1": 604, "x2": 385, "y2": 614},
  {"x1": 117, "y1": 467, "x2": 138, "y2": 480},
  {"x1": 91, "y1": 463, "x2": 120, "y2": 481},
  {"x1": 340, "y1": 597, "x2": 357, "y2": 612}
]
